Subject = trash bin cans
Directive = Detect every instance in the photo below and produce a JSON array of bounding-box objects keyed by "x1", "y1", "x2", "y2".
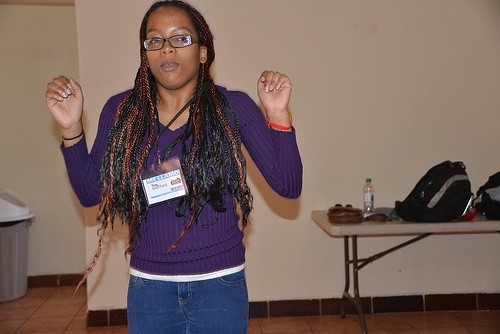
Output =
[{"x1": 1, "y1": 190, "x2": 37, "y2": 300}]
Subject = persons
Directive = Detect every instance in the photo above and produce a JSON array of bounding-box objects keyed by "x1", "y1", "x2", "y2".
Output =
[{"x1": 45, "y1": 0, "x2": 303, "y2": 334}]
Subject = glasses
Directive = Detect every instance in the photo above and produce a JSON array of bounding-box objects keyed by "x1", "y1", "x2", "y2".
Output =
[{"x1": 144, "y1": 35, "x2": 199, "y2": 51}]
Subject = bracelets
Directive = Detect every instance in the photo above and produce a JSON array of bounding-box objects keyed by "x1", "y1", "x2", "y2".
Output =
[
  {"x1": 61, "y1": 129, "x2": 84, "y2": 141},
  {"x1": 265, "y1": 111, "x2": 293, "y2": 130}
]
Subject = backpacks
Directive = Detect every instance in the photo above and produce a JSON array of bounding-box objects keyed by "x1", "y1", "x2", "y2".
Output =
[
  {"x1": 395, "y1": 160, "x2": 474, "y2": 222},
  {"x1": 472, "y1": 171, "x2": 500, "y2": 220}
]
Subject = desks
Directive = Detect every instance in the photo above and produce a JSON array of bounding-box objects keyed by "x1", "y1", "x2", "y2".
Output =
[{"x1": 312, "y1": 208, "x2": 500, "y2": 334}]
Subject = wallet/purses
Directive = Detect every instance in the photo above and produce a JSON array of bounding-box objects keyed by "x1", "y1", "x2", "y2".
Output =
[{"x1": 327, "y1": 207, "x2": 397, "y2": 223}]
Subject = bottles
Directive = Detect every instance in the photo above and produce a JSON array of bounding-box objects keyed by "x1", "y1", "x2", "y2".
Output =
[{"x1": 363, "y1": 178, "x2": 373, "y2": 216}]
